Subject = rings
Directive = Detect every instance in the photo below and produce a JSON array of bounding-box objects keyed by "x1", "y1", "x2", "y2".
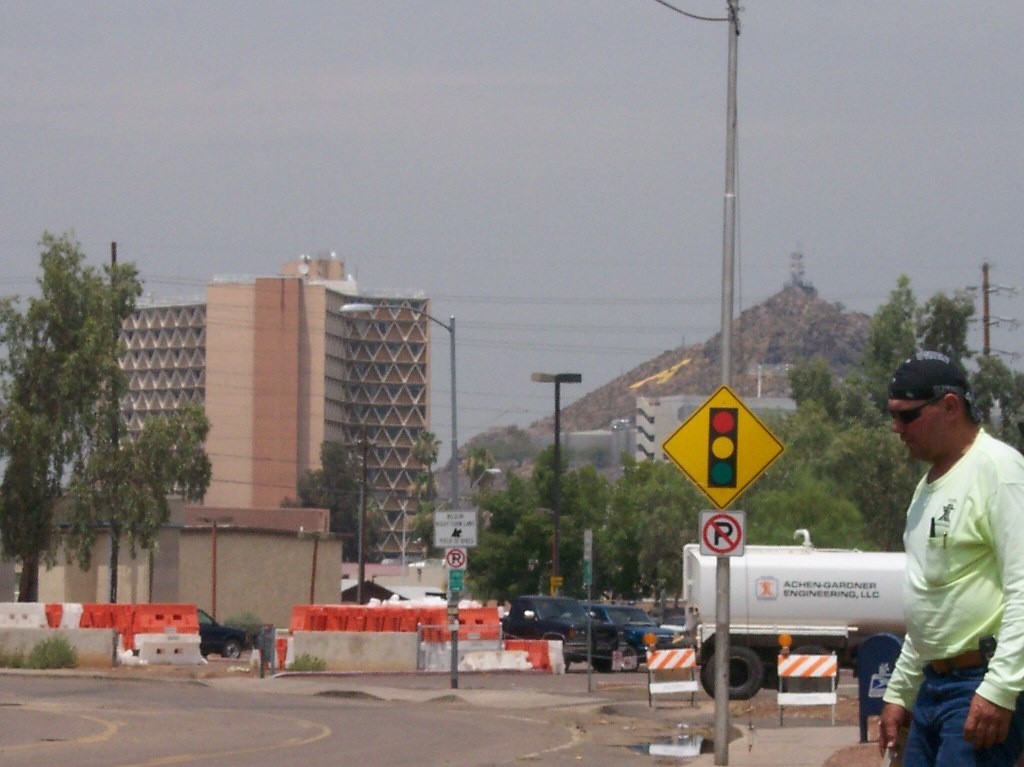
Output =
[{"x1": 879, "y1": 721, "x2": 885, "y2": 727}]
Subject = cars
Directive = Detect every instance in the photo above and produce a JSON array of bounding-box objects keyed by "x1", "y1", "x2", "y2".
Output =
[
  {"x1": 197, "y1": 608, "x2": 247, "y2": 659},
  {"x1": 504, "y1": 594, "x2": 579, "y2": 638}
]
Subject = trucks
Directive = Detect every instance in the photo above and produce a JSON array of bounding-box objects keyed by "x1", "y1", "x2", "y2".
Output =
[{"x1": 682, "y1": 527, "x2": 907, "y2": 701}]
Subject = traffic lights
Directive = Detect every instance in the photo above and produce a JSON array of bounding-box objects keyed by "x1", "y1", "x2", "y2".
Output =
[{"x1": 705, "y1": 403, "x2": 738, "y2": 489}]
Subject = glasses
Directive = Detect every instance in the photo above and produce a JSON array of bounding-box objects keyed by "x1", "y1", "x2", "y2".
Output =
[{"x1": 890, "y1": 399, "x2": 943, "y2": 424}]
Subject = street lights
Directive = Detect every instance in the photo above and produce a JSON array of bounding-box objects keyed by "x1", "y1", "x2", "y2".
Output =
[
  {"x1": 532, "y1": 372, "x2": 583, "y2": 597},
  {"x1": 340, "y1": 302, "x2": 459, "y2": 689}
]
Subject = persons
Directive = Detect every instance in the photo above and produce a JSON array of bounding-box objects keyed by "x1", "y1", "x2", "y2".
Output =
[{"x1": 878, "y1": 352, "x2": 1024, "y2": 767}]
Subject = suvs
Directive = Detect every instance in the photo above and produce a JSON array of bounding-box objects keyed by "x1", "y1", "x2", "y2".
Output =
[{"x1": 562, "y1": 603, "x2": 684, "y2": 675}]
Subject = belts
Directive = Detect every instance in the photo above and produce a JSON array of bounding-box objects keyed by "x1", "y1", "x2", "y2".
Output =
[{"x1": 931, "y1": 649, "x2": 983, "y2": 673}]
